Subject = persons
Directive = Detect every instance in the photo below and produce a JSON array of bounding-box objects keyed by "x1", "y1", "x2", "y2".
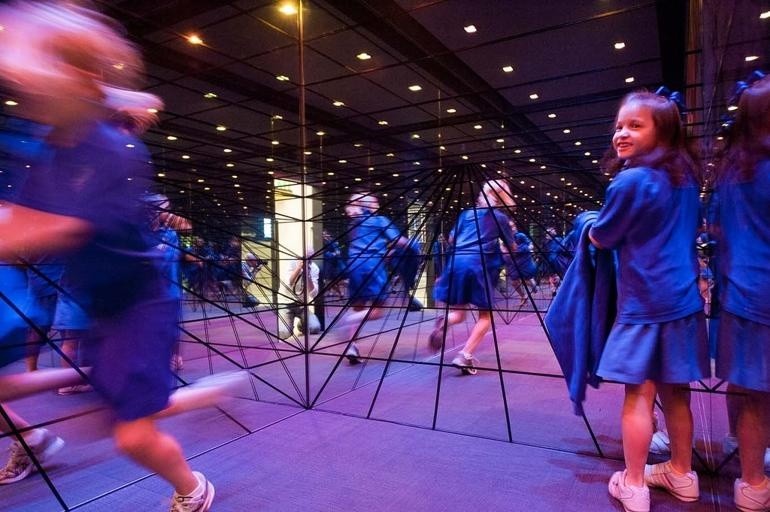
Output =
[
  {"x1": 698, "y1": 72, "x2": 770, "y2": 511},
  {"x1": 586, "y1": 83, "x2": 712, "y2": 511},
  {"x1": 0, "y1": 1, "x2": 216, "y2": 512},
  {"x1": 0, "y1": 121, "x2": 567, "y2": 484}
]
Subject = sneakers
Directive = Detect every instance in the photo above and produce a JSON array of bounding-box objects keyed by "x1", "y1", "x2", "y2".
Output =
[
  {"x1": 732, "y1": 474, "x2": 770, "y2": 511},
  {"x1": 168, "y1": 468, "x2": 216, "y2": 512},
  {"x1": 607, "y1": 461, "x2": 699, "y2": 512},
  {"x1": 452, "y1": 350, "x2": 479, "y2": 376},
  {"x1": 722, "y1": 429, "x2": 741, "y2": 460},
  {"x1": 0, "y1": 423, "x2": 66, "y2": 486},
  {"x1": 650, "y1": 427, "x2": 672, "y2": 458},
  {"x1": 345, "y1": 342, "x2": 363, "y2": 363},
  {"x1": 428, "y1": 315, "x2": 451, "y2": 355}
]
